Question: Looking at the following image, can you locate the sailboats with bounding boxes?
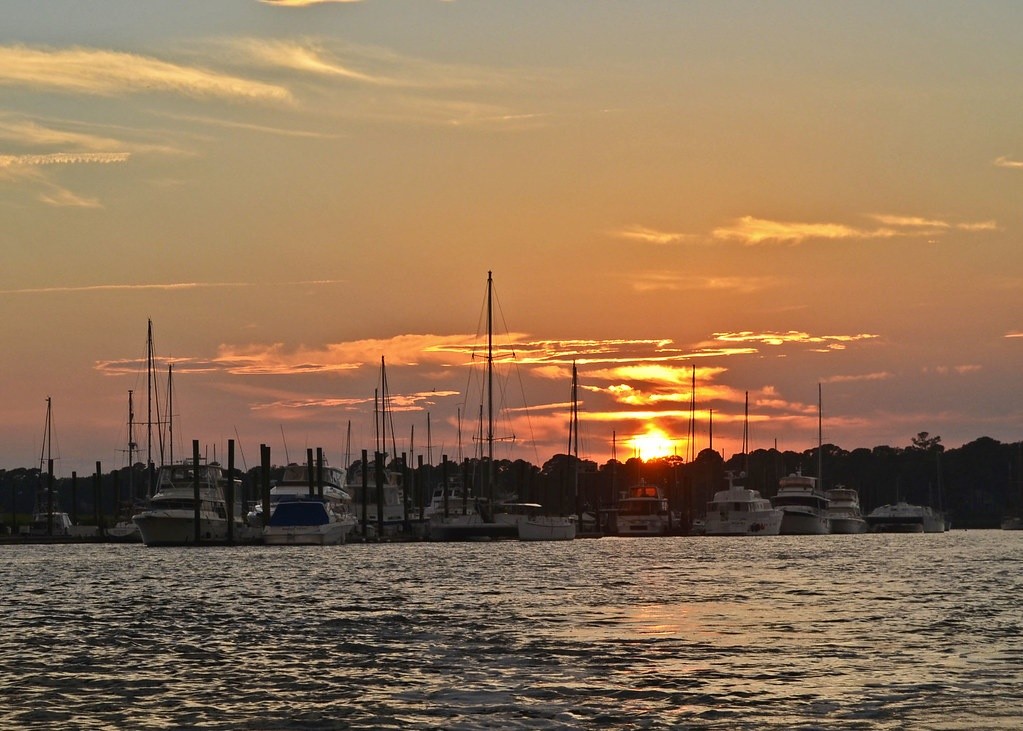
[104,270,950,548]
[19,397,99,536]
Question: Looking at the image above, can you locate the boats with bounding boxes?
[1001,516,1022,530]
